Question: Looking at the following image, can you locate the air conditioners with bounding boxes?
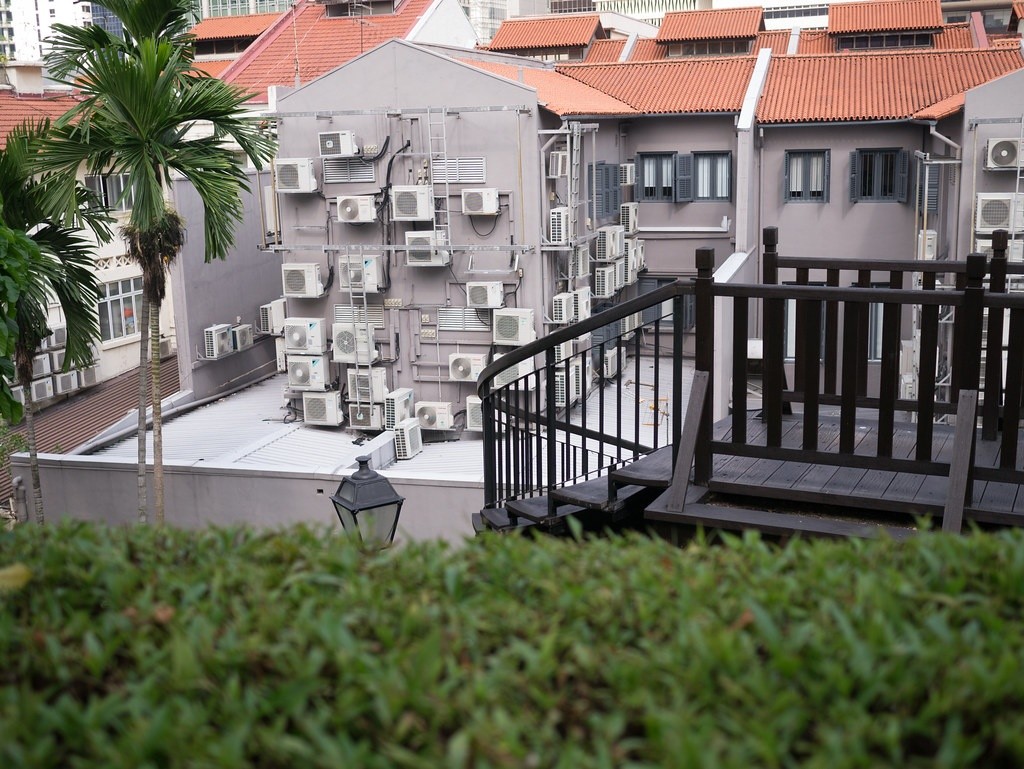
[899,191,1024,409]
[204,323,252,358]
[984,136,1023,168]
[4,323,103,406]
[159,337,172,360]
[336,195,378,223]
[258,301,384,431]
[390,184,435,221]
[264,158,317,237]
[461,187,501,215]
[404,230,450,266]
[385,281,535,459]
[281,262,324,300]
[339,254,385,293]
[317,131,357,158]
[544,150,647,409]
